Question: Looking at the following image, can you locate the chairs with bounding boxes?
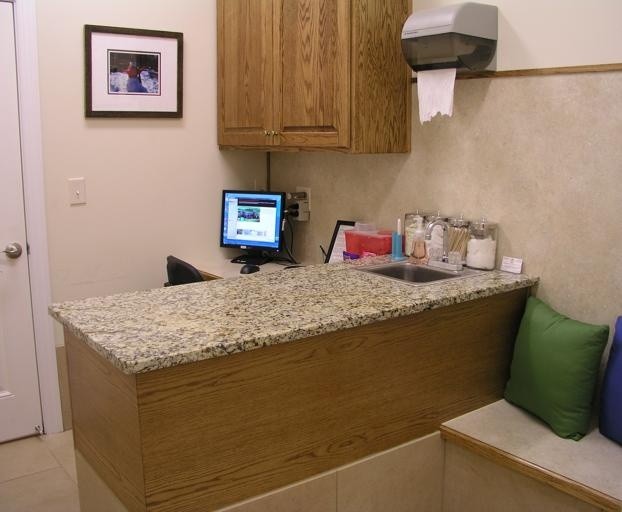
[166,256,204,283]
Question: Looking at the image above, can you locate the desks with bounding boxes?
[188,259,289,281]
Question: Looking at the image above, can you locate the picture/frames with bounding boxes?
[84,25,184,118]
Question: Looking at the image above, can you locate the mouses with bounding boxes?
[240,264,260,274]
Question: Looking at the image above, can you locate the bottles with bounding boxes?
[393,210,497,272]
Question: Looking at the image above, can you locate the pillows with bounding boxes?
[600,316,621,447]
[503,296,609,441]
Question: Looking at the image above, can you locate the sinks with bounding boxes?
[350,257,472,287]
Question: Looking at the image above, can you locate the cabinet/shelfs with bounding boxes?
[218,1,411,153]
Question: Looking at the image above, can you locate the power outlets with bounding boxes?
[294,189,311,225]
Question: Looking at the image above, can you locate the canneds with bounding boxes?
[405,210,497,271]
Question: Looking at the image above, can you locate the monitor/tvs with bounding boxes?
[220,190,286,265]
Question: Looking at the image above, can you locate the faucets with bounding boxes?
[424,217,464,272]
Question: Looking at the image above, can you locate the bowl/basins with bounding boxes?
[352,220,379,234]
